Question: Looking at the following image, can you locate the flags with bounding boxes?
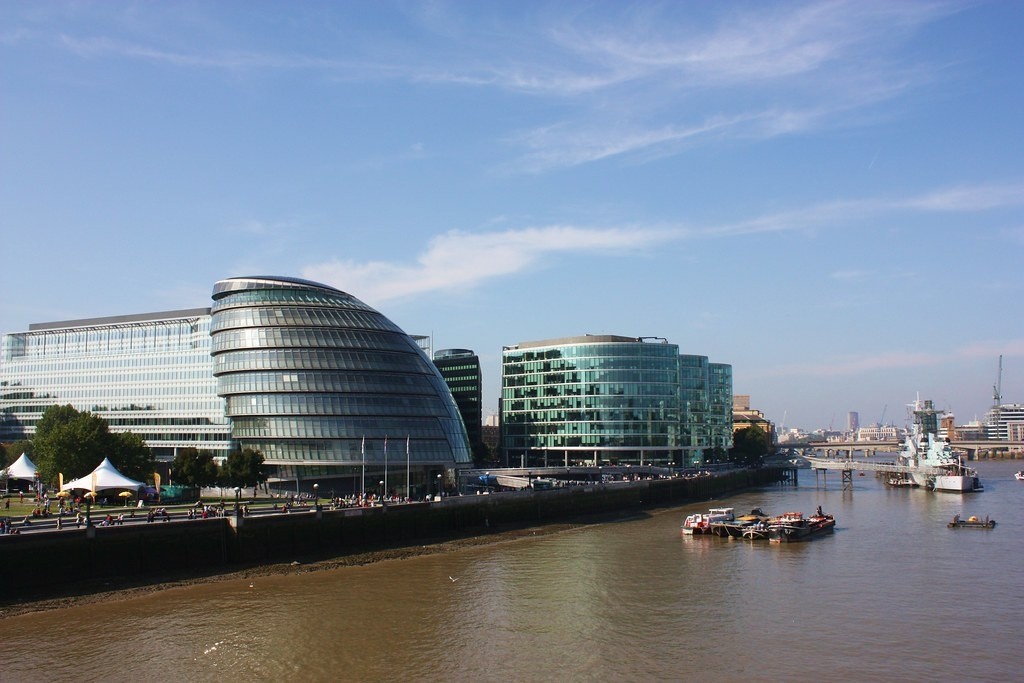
[406,436,410,454]
[361,437,365,454]
[383,437,387,454]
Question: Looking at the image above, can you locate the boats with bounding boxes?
[1014,470,1024,480]
[680,505,836,543]
[947,513,996,530]
[888,391,984,492]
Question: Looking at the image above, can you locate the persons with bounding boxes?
[0,486,530,535]
[539,461,711,487]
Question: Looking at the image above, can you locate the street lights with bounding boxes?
[528,470,532,488]
[599,466,602,482]
[668,462,675,475]
[437,473,442,495]
[313,484,319,510]
[234,487,240,512]
[627,465,631,479]
[648,463,652,476]
[486,472,489,491]
[86,493,91,528]
[379,481,385,504]
[694,455,763,471]
[567,468,570,484]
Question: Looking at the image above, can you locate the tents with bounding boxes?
[61,458,147,507]
[0,452,43,493]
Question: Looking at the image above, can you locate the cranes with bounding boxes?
[993,355,1002,405]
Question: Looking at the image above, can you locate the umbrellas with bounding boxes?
[84,492,98,505]
[56,492,70,507]
[141,487,167,506]
[118,491,133,507]
